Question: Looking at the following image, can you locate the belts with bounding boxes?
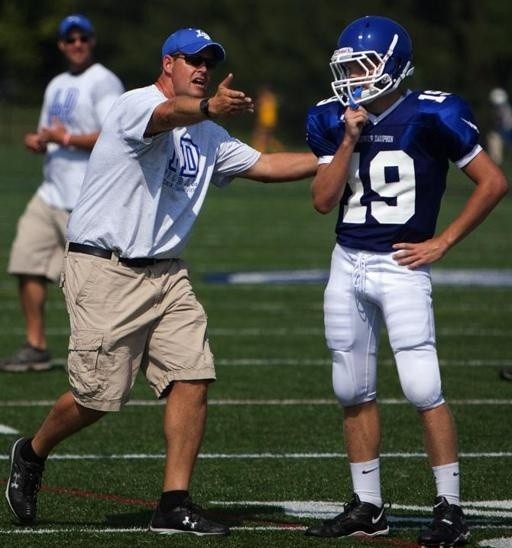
[68,241,166,268]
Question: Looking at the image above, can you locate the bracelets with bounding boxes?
[62,134,70,145]
[200,98,212,119]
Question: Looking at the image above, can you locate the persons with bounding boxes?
[303,15,509,546]
[4,25,316,535]
[487,87,511,169]
[0,15,128,373]
[251,87,284,156]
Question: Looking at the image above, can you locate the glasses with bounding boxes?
[176,54,216,69]
[65,37,89,43]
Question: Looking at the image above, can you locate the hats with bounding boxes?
[162,28,225,60]
[59,15,92,38]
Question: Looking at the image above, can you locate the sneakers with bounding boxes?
[6,435,45,521]
[305,493,392,540]
[418,497,471,548]
[1,342,52,372]
[150,496,231,537]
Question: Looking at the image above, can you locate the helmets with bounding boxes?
[329,16,416,108]
